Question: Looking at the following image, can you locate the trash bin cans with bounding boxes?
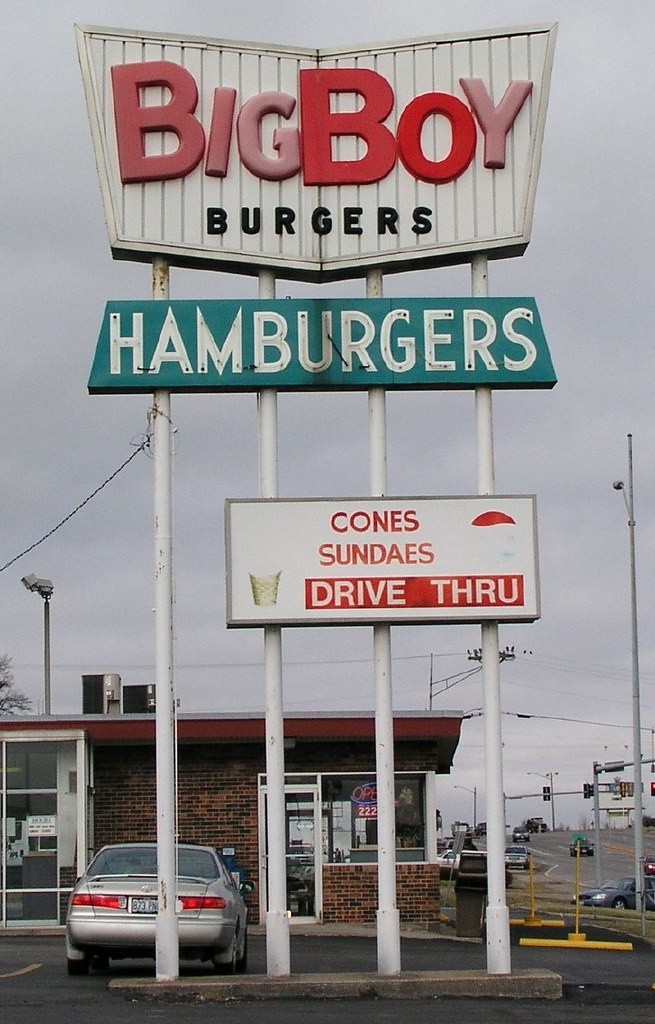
[452,856,486,937]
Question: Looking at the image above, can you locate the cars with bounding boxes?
[512,818,548,842]
[436,849,486,868]
[503,845,532,869]
[643,853,655,876]
[568,838,594,857]
[64,842,254,976]
[571,876,655,910]
[450,822,486,837]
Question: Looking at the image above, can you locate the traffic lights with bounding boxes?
[650,782,655,796]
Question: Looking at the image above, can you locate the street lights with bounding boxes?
[526,771,555,831]
[454,785,476,826]
[611,434,646,910]
